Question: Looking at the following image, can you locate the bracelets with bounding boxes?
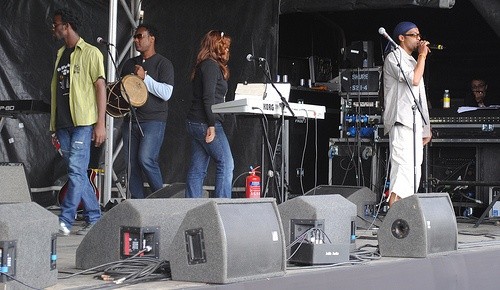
[418,53,426,59]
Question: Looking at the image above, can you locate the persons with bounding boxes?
[119,23,176,199]
[184,29,236,198]
[48,6,108,236]
[381,22,433,208]
[463,76,498,108]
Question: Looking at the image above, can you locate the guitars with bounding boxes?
[57,169,99,210]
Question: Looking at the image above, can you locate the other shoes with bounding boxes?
[76,223,95,235]
[59,222,72,236]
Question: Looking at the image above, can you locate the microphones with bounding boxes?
[426,44,447,50]
[246,53,265,62]
[378,26,399,49]
[96,36,110,45]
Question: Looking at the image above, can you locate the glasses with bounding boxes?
[133,33,151,38]
[402,32,422,38]
[52,22,66,28]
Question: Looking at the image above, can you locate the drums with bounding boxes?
[105,75,148,118]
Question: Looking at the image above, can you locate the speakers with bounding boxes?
[277,194,358,265]
[74,198,213,273]
[302,184,378,224]
[376,191,459,259]
[0,201,60,289]
[0,161,33,204]
[166,198,288,285]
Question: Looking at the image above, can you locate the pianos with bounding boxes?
[212,98,326,204]
[0,100,51,134]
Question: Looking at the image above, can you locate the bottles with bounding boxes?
[443,90,450,109]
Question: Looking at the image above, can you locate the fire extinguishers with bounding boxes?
[231,165,261,198]
